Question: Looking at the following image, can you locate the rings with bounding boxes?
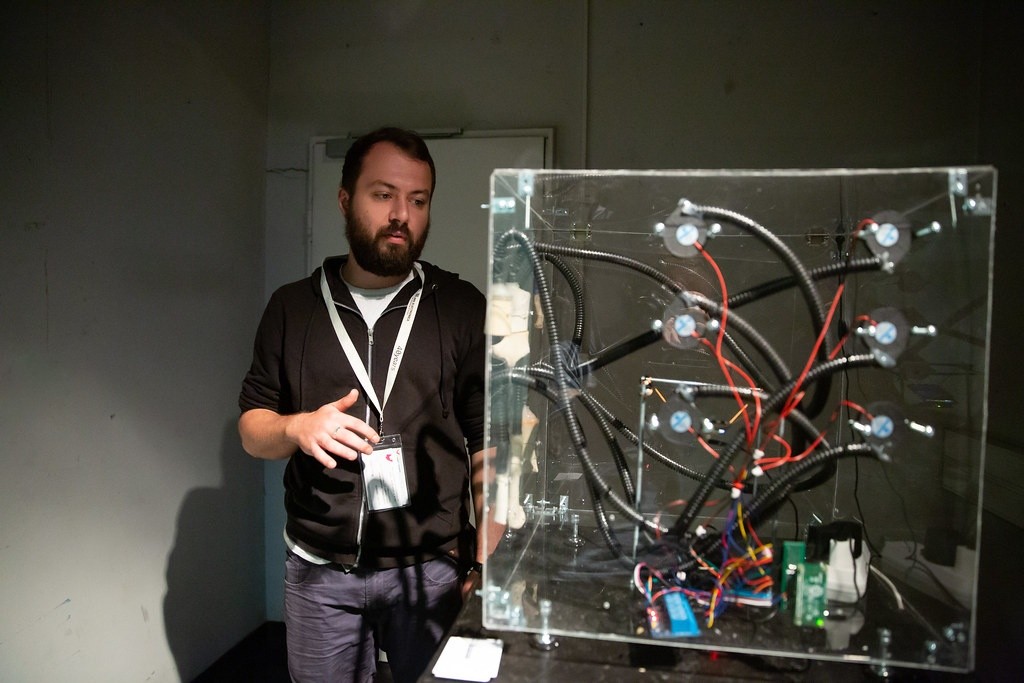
[334,426,340,439]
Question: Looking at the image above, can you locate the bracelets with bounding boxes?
[473,561,482,572]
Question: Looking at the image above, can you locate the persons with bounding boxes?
[238,128,507,683]
